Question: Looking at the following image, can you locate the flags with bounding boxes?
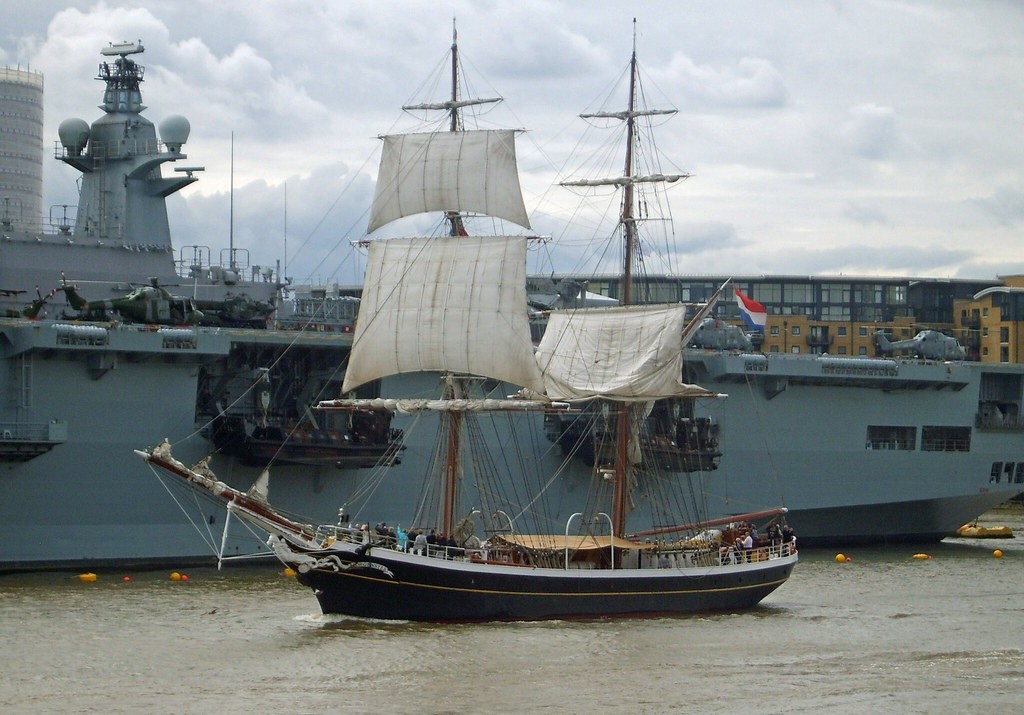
[734,285,767,335]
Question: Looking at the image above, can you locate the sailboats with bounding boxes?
[131,13,804,627]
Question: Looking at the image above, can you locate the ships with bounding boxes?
[0,19,1024,576]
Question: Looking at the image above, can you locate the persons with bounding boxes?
[720,520,797,566]
[353,521,466,559]
